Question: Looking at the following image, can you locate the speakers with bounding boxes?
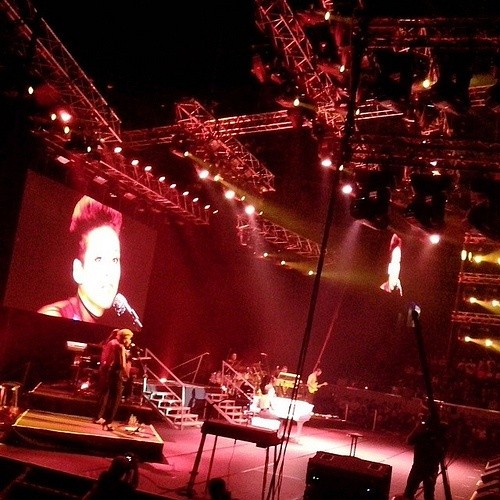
[305,451,392,500]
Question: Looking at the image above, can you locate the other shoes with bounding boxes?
[94,418,106,424]
[103,424,117,431]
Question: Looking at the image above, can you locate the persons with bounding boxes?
[94,330,133,431]
[38,195,120,325]
[402,411,448,500]
[224,352,321,406]
[379,234,408,299]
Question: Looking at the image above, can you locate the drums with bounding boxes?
[236,380,254,399]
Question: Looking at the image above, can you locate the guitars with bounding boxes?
[307,381,328,394]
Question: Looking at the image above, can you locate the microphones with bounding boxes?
[130,343,142,352]
[114,293,142,331]
[261,353,267,356]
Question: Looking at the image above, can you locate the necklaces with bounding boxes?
[77,291,103,322]
